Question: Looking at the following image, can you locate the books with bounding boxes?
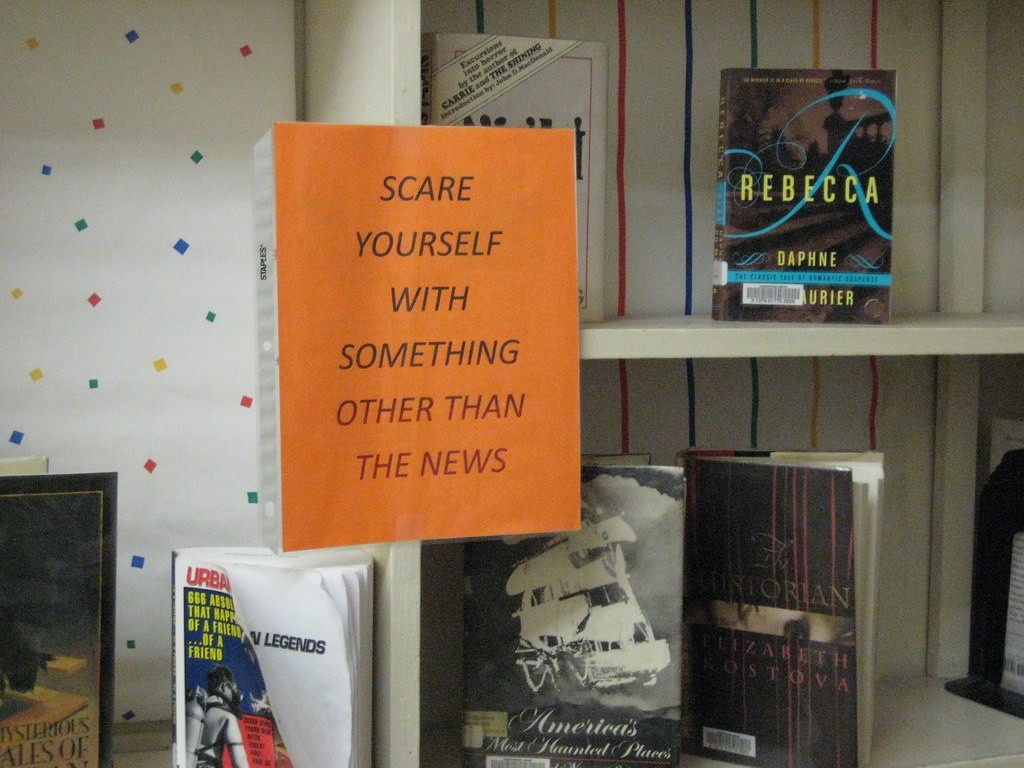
[458,453,685,768]
[420,31,604,321]
[711,69,897,325]
[172,547,374,768]
[675,450,885,768]
[0,457,118,768]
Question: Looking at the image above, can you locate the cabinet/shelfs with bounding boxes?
[296,1,1024,768]
